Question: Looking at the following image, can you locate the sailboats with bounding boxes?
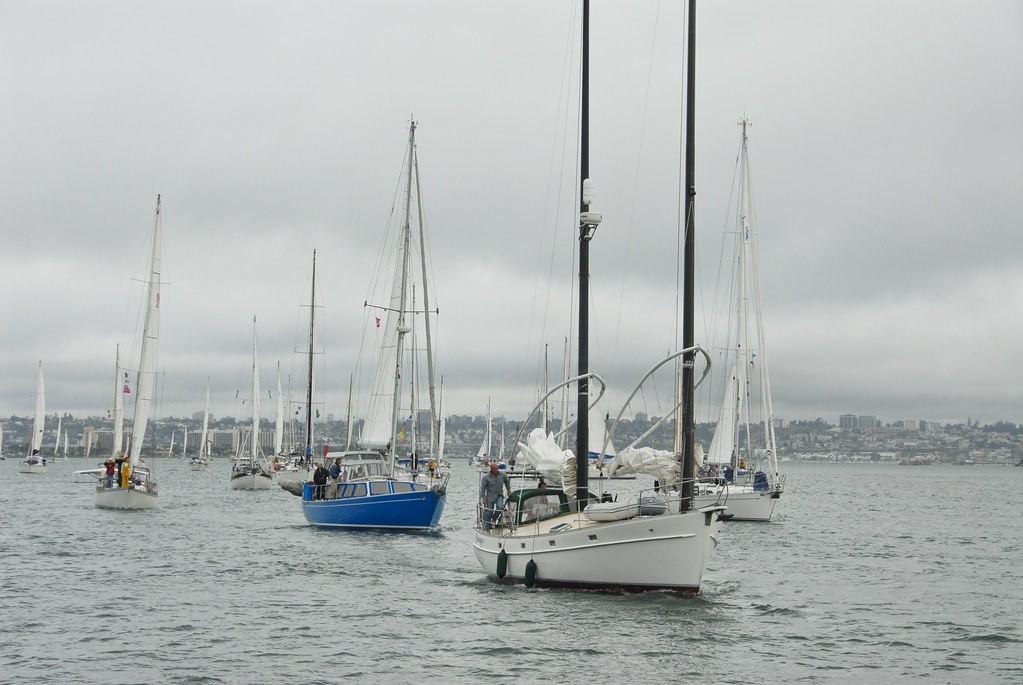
[301,112,455,533]
[43,416,94,464]
[95,195,167,511]
[19,359,47,474]
[229,314,299,492]
[474,0,734,596]
[655,113,785,522]
[271,246,335,499]
[177,428,188,462]
[468,395,512,469]
[190,376,213,472]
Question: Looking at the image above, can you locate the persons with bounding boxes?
[410,452,418,470]
[314,462,329,500]
[326,458,342,499]
[532,496,558,518]
[479,463,512,530]
[740,459,745,470]
[305,444,311,461]
[104,453,130,488]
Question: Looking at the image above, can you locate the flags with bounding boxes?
[316,410,320,418]
[376,317,380,328]
[124,385,130,393]
